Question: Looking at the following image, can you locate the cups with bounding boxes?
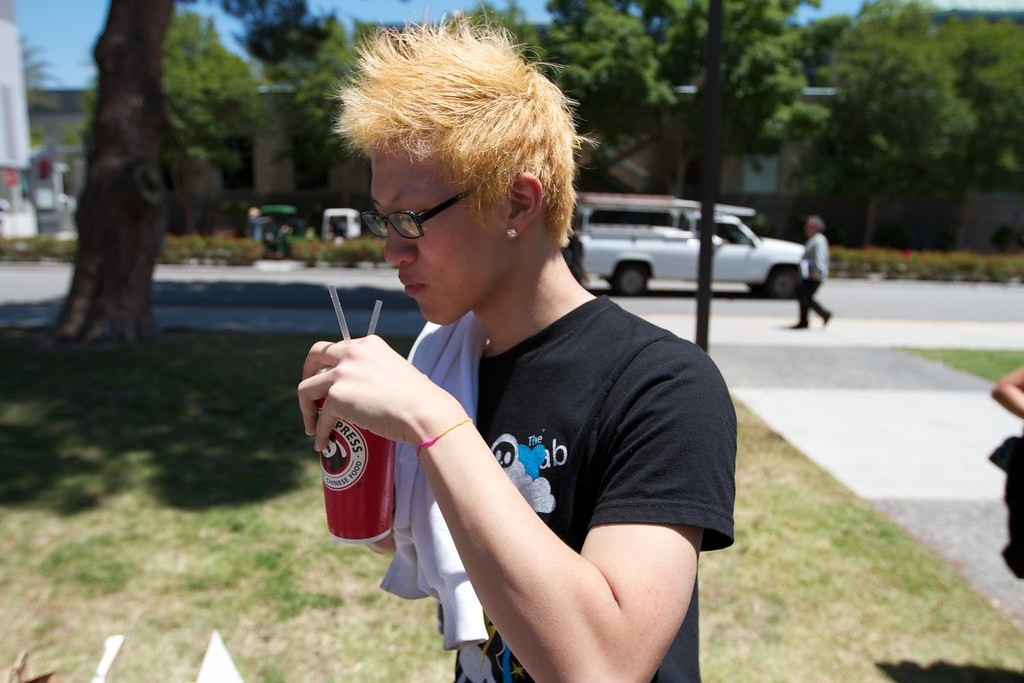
[313,367,396,543]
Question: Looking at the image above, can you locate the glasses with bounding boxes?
[361,188,480,240]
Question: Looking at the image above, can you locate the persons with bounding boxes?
[990,365,1024,418]
[792,215,833,329]
[296,9,739,683]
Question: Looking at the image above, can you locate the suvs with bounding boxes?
[566,193,811,297]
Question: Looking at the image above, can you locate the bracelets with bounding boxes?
[415,418,473,461]
